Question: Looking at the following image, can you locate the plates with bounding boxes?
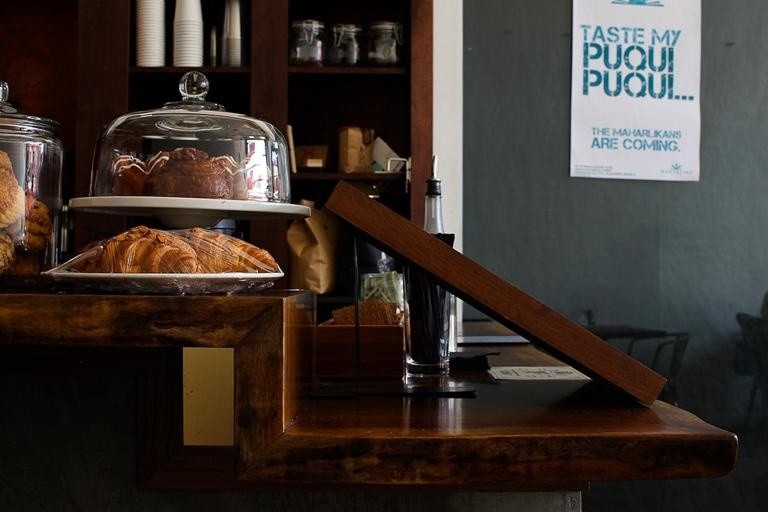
[41,266,289,296]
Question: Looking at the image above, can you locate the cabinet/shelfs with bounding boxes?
[75,1,432,304]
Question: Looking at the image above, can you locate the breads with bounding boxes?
[0,150,57,273]
[72,226,279,274]
[100,147,250,201]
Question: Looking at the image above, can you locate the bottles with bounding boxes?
[0,82,65,291]
[292,21,403,66]
[413,176,464,360]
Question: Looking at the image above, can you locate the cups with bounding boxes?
[135,1,243,69]
[401,263,452,378]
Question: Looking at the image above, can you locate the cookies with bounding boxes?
[333,301,401,325]
